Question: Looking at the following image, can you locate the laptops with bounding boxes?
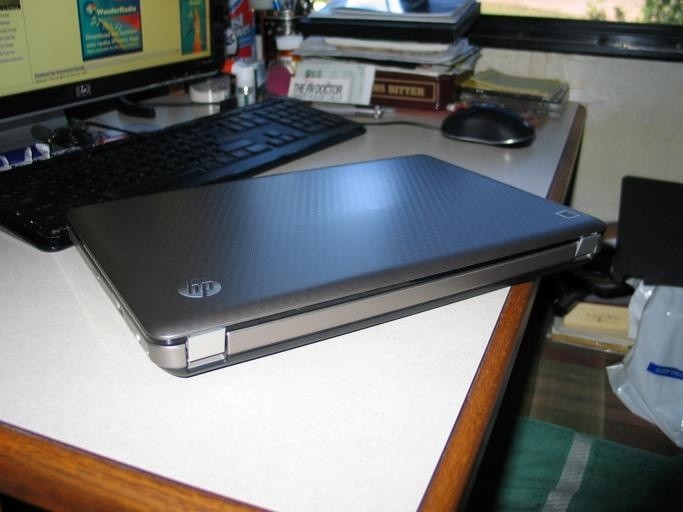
[64,152,607,379]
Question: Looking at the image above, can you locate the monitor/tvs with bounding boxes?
[0,0,227,149]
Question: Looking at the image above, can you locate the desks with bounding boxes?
[0,80,589,512]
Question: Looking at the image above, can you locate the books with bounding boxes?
[289,37,481,78]
[459,66,571,120]
[545,301,634,356]
[307,0,478,23]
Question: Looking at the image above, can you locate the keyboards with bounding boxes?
[0,95,367,253]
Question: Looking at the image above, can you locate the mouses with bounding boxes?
[439,105,537,150]
